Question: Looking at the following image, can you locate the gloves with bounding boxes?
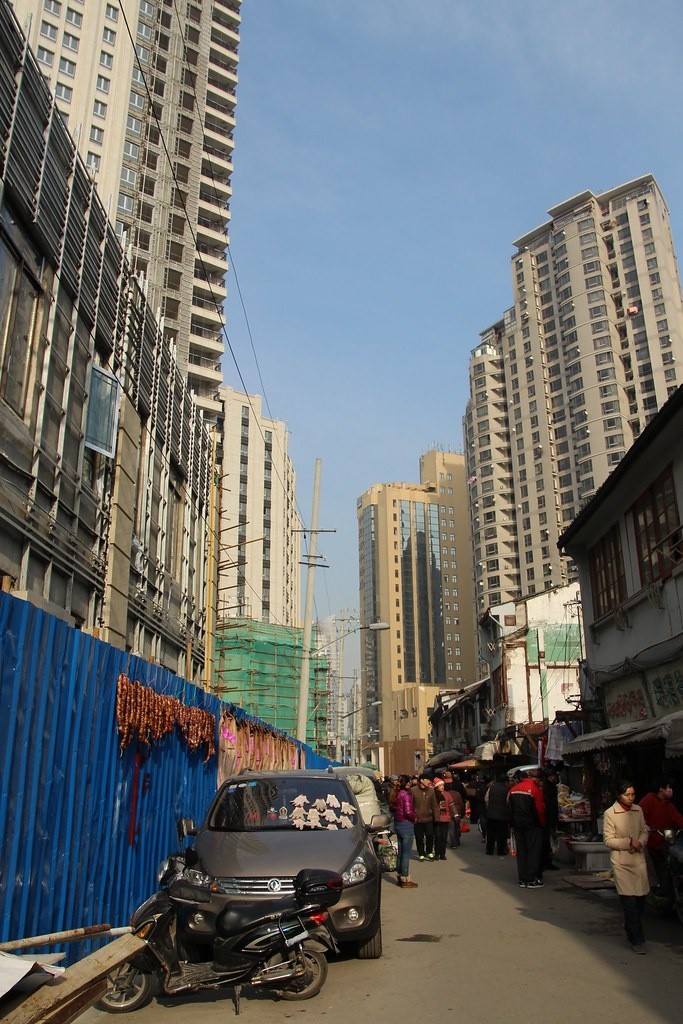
[455,816,460,824]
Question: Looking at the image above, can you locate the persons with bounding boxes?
[638,776,683,898]
[393,776,419,888]
[381,772,468,862]
[468,768,546,888]
[600,783,650,955]
[539,768,562,871]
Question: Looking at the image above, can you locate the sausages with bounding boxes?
[117,674,215,766]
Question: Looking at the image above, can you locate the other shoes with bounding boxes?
[435,855,439,860]
[527,879,544,888]
[631,944,647,953]
[427,853,433,858]
[440,856,446,860]
[419,856,425,859]
[481,837,486,843]
[518,881,527,887]
[546,864,559,870]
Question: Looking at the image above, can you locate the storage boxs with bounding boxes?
[569,840,611,871]
[598,819,603,834]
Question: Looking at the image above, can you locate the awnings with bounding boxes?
[561,709,683,768]
[447,757,495,769]
[474,740,511,761]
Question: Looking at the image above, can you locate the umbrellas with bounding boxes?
[424,748,464,769]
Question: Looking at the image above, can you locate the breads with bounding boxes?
[557,796,576,807]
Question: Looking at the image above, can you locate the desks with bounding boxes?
[560,817,593,835]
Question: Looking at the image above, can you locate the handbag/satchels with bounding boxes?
[550,836,560,857]
[459,818,471,833]
[507,830,518,857]
[465,782,477,797]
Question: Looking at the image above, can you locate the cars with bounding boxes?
[327,766,396,835]
[177,764,391,960]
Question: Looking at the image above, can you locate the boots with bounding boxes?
[397,875,400,886]
[400,875,418,888]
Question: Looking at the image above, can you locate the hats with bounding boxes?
[434,777,444,788]
[530,769,542,778]
[419,773,429,779]
[441,772,452,779]
[412,775,417,780]
[390,775,398,782]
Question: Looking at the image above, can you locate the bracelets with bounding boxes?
[630,838,633,846]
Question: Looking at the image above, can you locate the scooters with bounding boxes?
[95,846,346,1015]
[644,828,683,924]
